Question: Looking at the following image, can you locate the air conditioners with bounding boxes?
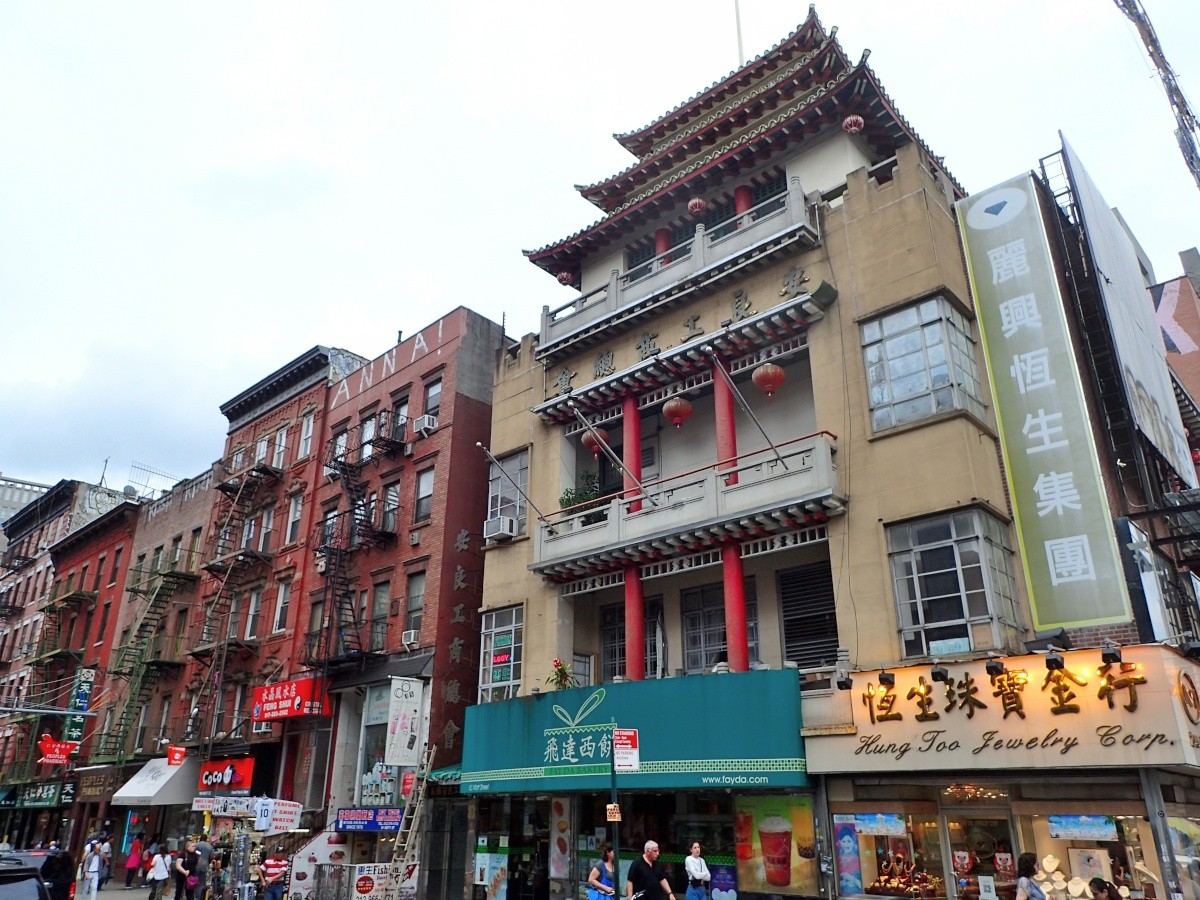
[414,414,436,433]
[484,516,518,540]
[253,721,272,733]
[402,630,419,645]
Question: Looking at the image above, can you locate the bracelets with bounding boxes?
[667,892,672,896]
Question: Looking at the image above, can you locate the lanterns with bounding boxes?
[842,112,864,135]
[687,197,706,217]
[662,397,692,432]
[751,364,785,400]
[582,428,607,460]
[557,272,573,285]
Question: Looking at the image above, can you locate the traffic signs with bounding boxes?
[613,729,640,771]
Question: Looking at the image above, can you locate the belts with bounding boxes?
[88,871,99,873]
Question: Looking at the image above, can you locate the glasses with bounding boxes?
[650,849,660,853]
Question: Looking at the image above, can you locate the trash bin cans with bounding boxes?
[239,884,255,900]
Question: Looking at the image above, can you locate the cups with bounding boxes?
[790,795,817,858]
[758,831,793,886]
[735,809,753,859]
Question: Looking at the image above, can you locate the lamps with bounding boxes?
[879,669,894,684]
[1045,649,1064,669]
[721,319,732,327]
[1102,643,1122,663]
[986,658,1003,675]
[837,674,852,690]
[650,348,661,356]
[931,663,948,682]
[1180,642,1200,656]
[564,387,573,394]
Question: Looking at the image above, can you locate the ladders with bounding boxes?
[380,742,439,900]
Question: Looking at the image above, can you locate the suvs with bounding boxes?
[0,849,76,900]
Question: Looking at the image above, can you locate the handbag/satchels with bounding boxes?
[146,867,156,883]
[142,849,149,861]
[186,876,200,890]
[137,865,144,877]
[690,879,702,887]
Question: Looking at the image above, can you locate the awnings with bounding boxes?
[111,756,201,806]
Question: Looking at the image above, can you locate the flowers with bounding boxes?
[545,658,580,691]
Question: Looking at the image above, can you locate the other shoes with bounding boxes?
[141,884,151,888]
[124,887,133,889]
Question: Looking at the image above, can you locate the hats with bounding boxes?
[276,846,285,852]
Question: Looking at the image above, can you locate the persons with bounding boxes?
[258,846,289,900]
[1087,878,1123,900]
[1015,852,1046,900]
[32,831,216,900]
[684,839,712,900]
[626,840,676,900]
[588,842,616,900]
[836,825,861,891]
